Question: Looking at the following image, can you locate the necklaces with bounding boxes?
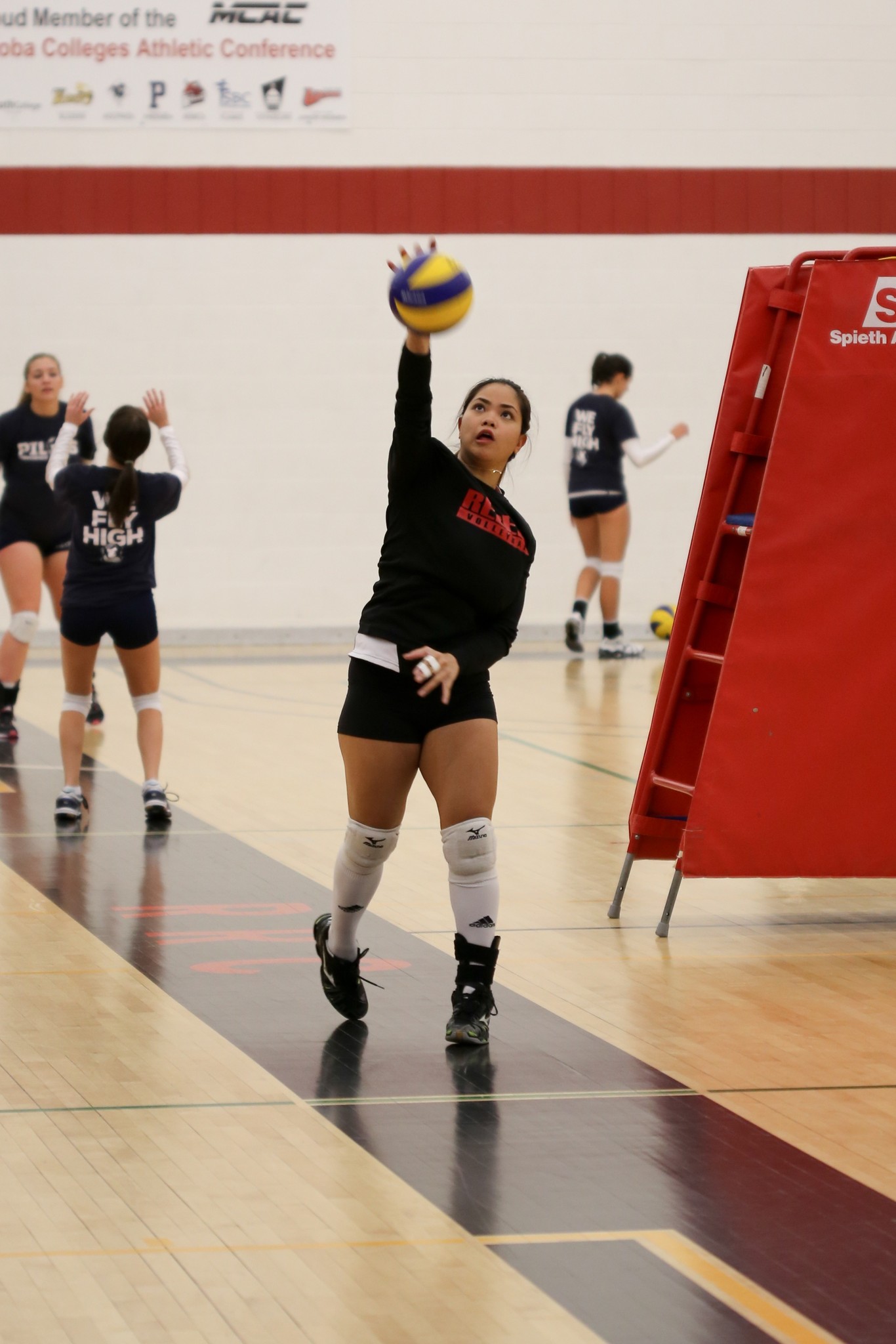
[468,465,503,475]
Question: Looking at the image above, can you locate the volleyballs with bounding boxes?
[650,604,677,640]
[389,250,473,334]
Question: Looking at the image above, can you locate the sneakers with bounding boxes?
[54,818,90,844]
[445,1042,499,1117]
[444,985,502,1045]
[564,609,586,654]
[313,913,385,1019]
[313,1019,369,1106]
[84,672,104,723]
[53,785,90,820]
[598,633,648,659]
[143,826,171,853]
[0,703,18,738]
[144,783,180,819]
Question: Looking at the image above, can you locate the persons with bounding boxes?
[46,388,187,821]
[564,352,689,660]
[313,237,538,1043]
[0,352,97,740]
